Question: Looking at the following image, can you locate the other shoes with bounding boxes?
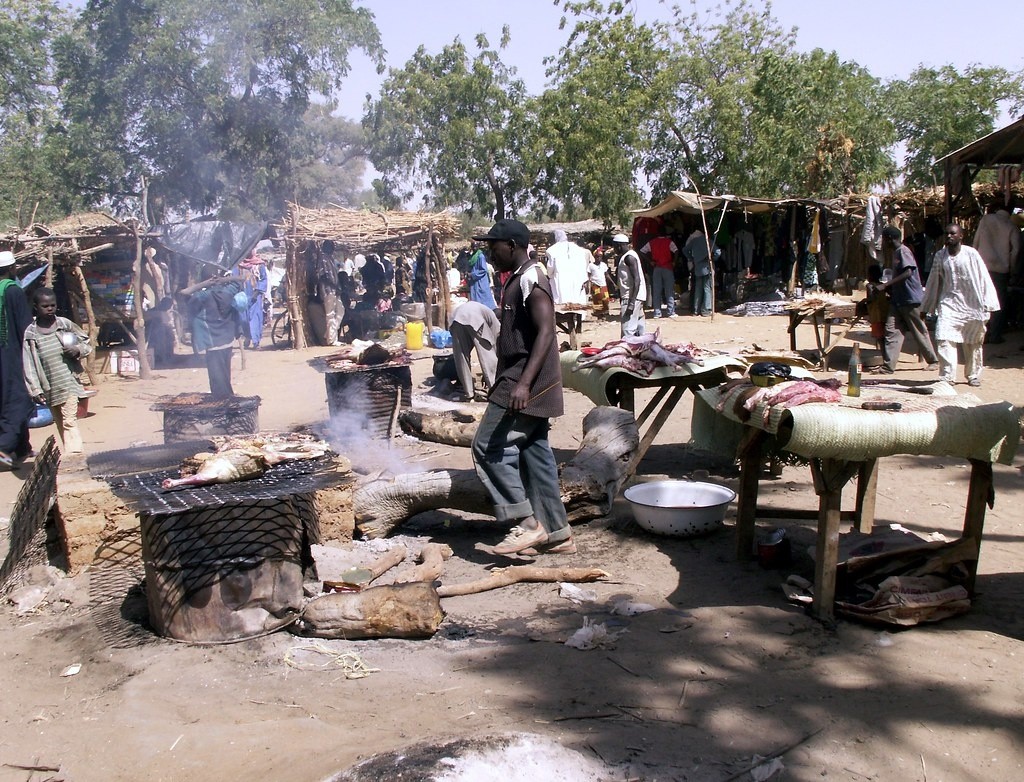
[668,314,678,317]
[702,310,713,317]
[925,363,938,371]
[654,313,662,319]
[871,366,893,375]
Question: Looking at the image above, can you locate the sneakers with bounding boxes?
[516,535,577,555]
[491,520,549,555]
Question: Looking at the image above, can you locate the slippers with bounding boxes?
[0,456,21,469]
[18,450,38,461]
[948,380,956,386]
[968,378,980,387]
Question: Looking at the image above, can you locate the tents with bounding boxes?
[629,191,866,322]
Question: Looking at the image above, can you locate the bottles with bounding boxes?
[766,527,785,543]
[847,343,863,397]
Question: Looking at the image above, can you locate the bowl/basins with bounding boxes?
[623,479,736,535]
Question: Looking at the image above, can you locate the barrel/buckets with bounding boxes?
[405,321,425,350]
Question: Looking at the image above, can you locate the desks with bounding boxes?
[568,339,801,493]
[717,391,1014,628]
[782,298,922,372]
[556,304,595,349]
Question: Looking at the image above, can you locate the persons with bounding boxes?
[449,300,502,399]
[0,251,34,469]
[22,287,92,452]
[231,247,268,350]
[144,296,176,356]
[863,265,890,353]
[613,232,646,339]
[639,227,679,319]
[869,227,939,375]
[920,223,1001,386]
[527,229,619,334]
[973,204,1021,345]
[682,229,721,317]
[472,219,577,555]
[180,265,246,394]
[316,239,498,346]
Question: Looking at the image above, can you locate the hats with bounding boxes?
[471,219,530,248]
[0,251,15,267]
[613,234,629,242]
[593,249,602,256]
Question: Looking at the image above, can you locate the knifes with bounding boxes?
[822,402,902,409]
[861,385,933,394]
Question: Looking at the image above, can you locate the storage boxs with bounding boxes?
[111,345,154,373]
[77,271,134,313]
